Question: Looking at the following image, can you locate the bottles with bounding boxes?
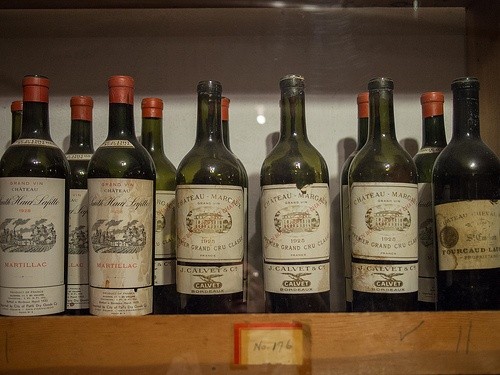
[0,72,500,317]
